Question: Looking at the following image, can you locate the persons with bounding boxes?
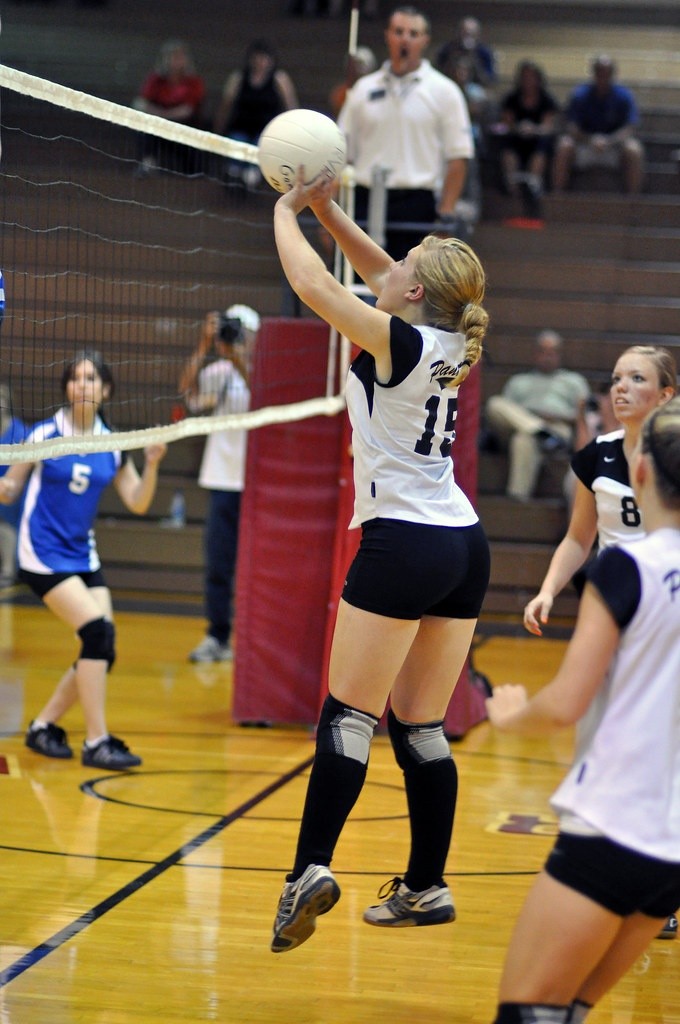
[270,164,491,954]
[132,16,645,240]
[318,6,475,308]
[484,324,680,1024]
[0,237,346,771]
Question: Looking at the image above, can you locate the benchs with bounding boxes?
[0,79,680,619]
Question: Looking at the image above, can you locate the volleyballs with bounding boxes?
[259,107,349,194]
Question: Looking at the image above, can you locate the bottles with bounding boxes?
[170,487,186,529]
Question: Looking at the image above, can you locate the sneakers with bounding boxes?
[364,877,455,927]
[80,733,142,768]
[657,914,677,938]
[25,720,73,758]
[270,864,340,953]
[190,636,233,663]
